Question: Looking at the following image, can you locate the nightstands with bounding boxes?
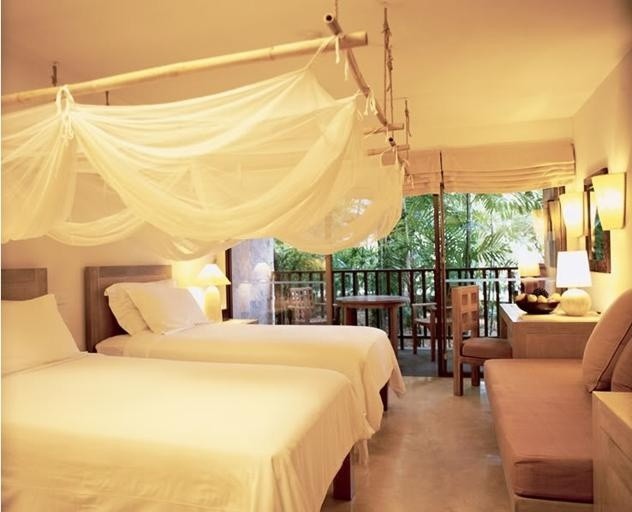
[222,316,260,325]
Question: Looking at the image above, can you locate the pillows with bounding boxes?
[581,284,631,391]
[4,293,81,378]
[610,336,631,390]
[103,280,180,336]
[122,280,209,334]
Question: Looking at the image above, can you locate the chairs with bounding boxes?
[451,285,511,396]
[287,287,341,324]
[410,283,460,360]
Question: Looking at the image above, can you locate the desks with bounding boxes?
[334,295,412,362]
[499,303,604,359]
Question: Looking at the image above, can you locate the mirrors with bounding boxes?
[583,168,610,273]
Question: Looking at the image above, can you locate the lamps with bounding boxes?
[589,172,625,230]
[557,192,583,238]
[518,251,545,294]
[554,250,593,316]
[530,207,551,245]
[176,264,233,324]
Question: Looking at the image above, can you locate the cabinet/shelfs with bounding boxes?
[591,389,631,511]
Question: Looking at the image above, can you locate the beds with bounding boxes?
[2,266,375,510]
[84,263,407,465]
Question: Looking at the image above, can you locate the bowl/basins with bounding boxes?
[514,301,560,315]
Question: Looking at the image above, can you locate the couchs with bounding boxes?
[482,286,632,511]
